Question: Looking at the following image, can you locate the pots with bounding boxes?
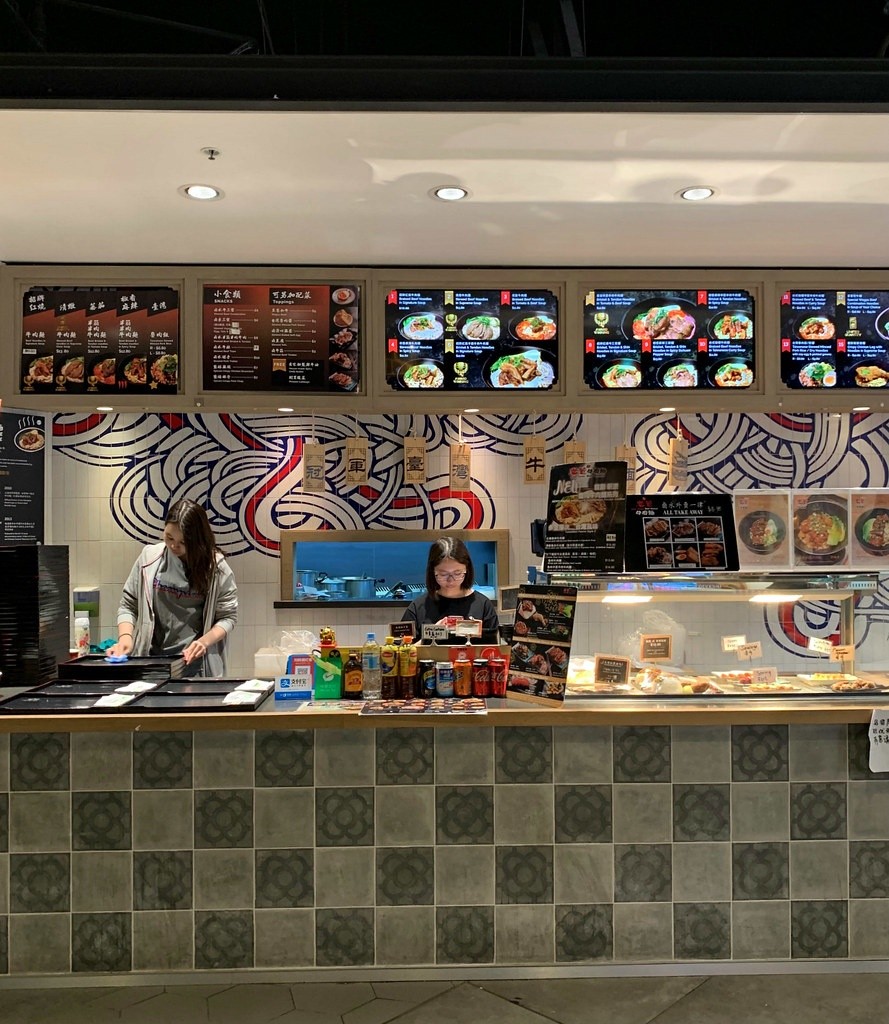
[297,570,385,600]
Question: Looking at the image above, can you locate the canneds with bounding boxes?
[416,658,508,698]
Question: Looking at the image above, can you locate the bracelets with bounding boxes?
[118,633,133,641]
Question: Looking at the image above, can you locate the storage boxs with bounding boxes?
[252,647,289,678]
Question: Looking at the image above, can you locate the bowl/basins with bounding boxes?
[595,358,641,388]
[874,307,889,341]
[794,500,848,557]
[854,507,889,556]
[792,310,836,341]
[846,358,889,388]
[508,310,557,341]
[707,309,754,339]
[707,356,754,387]
[481,345,558,388]
[739,510,786,556]
[23,352,179,390]
[798,360,836,387]
[621,296,697,340]
[656,357,698,387]
[398,311,445,341]
[455,310,502,341]
[396,358,444,389]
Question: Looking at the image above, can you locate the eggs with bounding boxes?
[822,372,837,387]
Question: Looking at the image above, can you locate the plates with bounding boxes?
[329,372,357,391]
[797,673,859,686]
[14,427,46,453]
[330,328,357,350]
[332,288,355,305]
[334,314,353,327]
[329,352,357,369]
[511,642,534,662]
[545,646,568,670]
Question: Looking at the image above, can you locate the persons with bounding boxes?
[401,537,499,645]
[106,499,240,678]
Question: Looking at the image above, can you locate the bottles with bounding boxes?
[361,632,382,700]
[342,654,364,700]
[398,636,418,699]
[380,636,399,700]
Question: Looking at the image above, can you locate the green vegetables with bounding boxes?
[156,354,178,374]
[802,317,830,327]
[763,518,778,547]
[827,516,846,546]
[806,361,835,378]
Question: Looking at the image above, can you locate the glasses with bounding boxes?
[434,569,467,581]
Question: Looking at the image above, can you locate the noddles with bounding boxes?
[602,304,754,387]
[403,313,557,389]
